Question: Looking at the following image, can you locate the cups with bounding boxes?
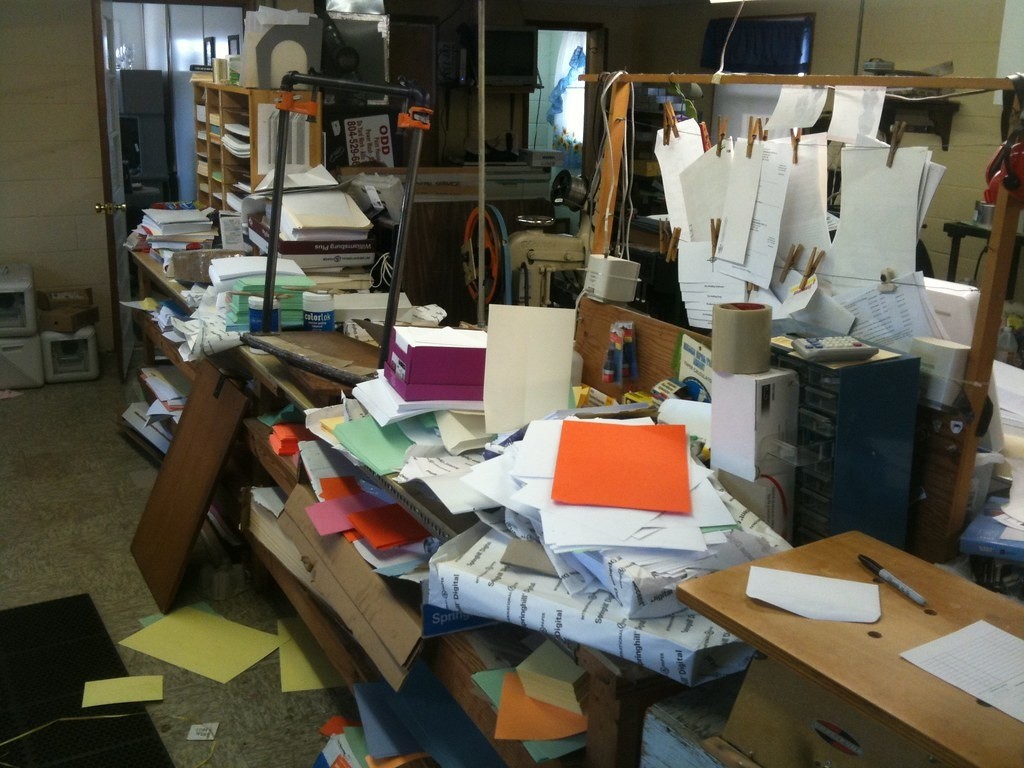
[212,58,227,83]
[711,302,771,375]
[228,55,241,85]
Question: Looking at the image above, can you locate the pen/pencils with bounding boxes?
[856,553,928,606]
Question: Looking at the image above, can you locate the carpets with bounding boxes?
[0,593,177,768]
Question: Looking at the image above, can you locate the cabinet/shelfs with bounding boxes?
[127,245,683,768]
[631,294,921,547]
[194,80,322,210]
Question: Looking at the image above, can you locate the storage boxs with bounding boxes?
[383,324,488,401]
[36,286,100,332]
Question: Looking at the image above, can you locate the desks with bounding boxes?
[675,529,1024,768]
[944,219,1024,300]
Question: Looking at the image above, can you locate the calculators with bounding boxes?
[788,335,880,363]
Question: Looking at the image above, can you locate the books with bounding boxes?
[516,639,590,718]
[122,100,373,429]
